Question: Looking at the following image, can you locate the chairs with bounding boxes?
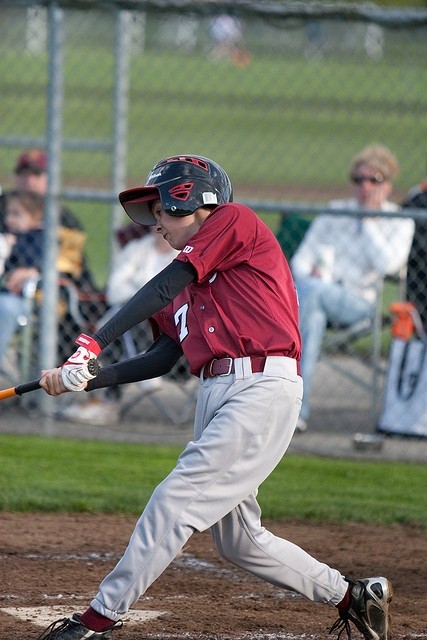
[273,212,388,413]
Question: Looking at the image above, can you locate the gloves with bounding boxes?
[61,333,102,392]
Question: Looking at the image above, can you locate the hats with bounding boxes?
[352,146,396,178]
[15,153,47,175]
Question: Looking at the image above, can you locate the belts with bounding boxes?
[203,357,302,380]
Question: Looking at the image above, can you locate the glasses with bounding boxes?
[351,176,384,185]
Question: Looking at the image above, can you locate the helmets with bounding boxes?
[119,155,232,226]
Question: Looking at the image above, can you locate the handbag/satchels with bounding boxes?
[378,340,426,436]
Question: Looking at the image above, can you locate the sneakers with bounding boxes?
[329,574,393,640]
[37,613,123,640]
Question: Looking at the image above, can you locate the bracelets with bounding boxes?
[75,333,101,356]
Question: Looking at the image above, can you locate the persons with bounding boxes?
[34,154,394,640]
[0,147,124,429]
[290,141,417,432]
[105,218,201,425]
[0,188,96,335]
